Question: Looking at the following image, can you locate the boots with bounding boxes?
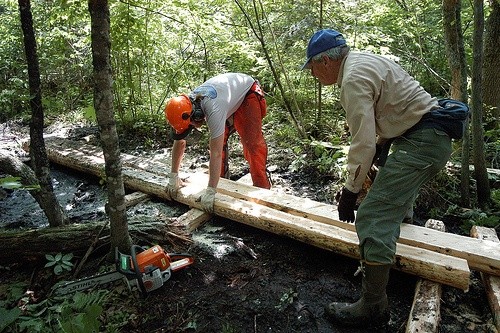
[326,260,391,325]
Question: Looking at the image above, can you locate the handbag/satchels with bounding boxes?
[432,98,469,140]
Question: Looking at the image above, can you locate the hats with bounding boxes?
[301,29,346,71]
[165,93,196,140]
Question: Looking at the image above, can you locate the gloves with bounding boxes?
[370,143,383,166]
[164,173,187,199]
[335,186,359,223]
[195,187,217,214]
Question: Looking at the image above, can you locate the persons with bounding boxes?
[301,28,452,323]
[165,72,272,214]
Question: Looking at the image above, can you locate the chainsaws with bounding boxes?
[53,244,193,297]
